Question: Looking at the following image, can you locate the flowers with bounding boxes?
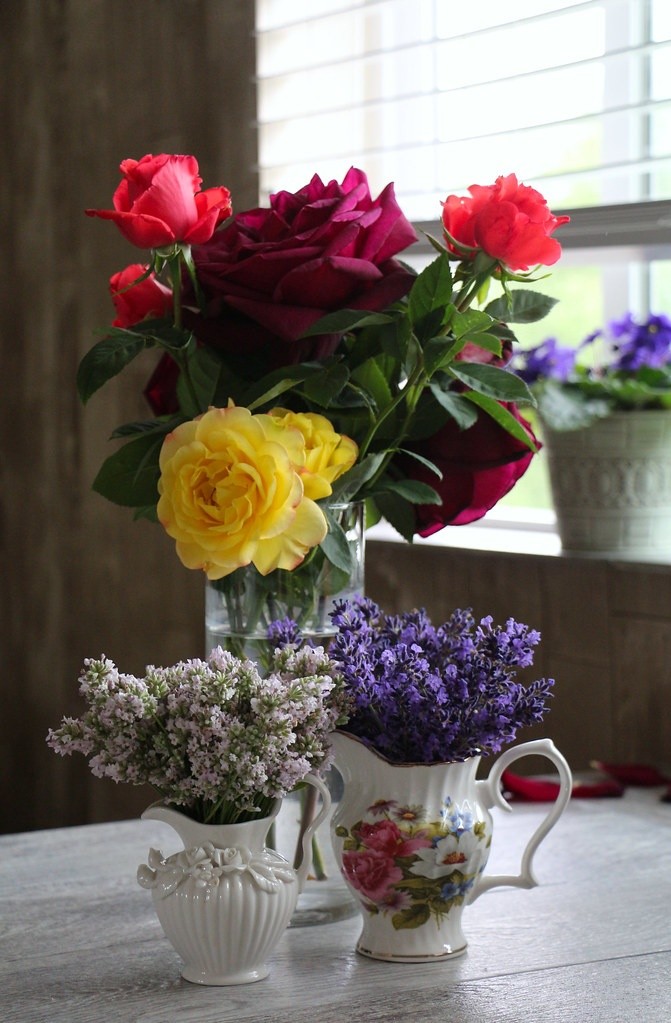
[507,313,671,430]
[77,155,568,875]
[265,596,554,766]
[47,643,350,823]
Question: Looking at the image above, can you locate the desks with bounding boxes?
[0,769,670,1023]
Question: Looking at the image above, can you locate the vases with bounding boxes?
[136,775,330,988]
[204,500,372,928]
[317,730,572,963]
[546,410,671,552]
